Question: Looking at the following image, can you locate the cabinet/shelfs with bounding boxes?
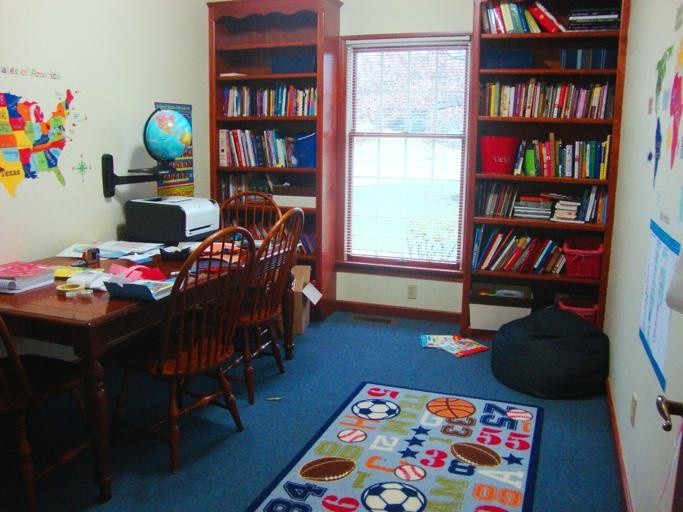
[458,1,632,341]
[206,0,342,322]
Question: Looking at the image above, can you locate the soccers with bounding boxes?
[353,398,400,420]
[361,482,427,512]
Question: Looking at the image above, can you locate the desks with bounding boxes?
[0,240,305,502]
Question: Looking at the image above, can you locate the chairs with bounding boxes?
[114,225,260,475]
[227,206,306,405]
[219,192,283,245]
[2,318,115,510]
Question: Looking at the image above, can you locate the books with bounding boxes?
[514,132,613,179]
[421,334,462,349]
[472,281,532,299]
[0,219,316,303]
[218,175,316,208]
[222,82,317,117]
[481,4,621,34]
[479,77,614,120]
[217,127,315,169]
[472,181,609,275]
[441,337,490,357]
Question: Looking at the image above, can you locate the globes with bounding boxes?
[143,108,192,175]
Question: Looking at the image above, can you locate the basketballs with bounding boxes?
[429,398,475,420]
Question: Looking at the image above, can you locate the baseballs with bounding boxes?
[507,409,533,422]
[395,464,426,481]
[338,428,368,443]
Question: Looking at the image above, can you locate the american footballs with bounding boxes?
[452,442,500,466]
[298,457,355,481]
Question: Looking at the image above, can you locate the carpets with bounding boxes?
[244,381,544,511]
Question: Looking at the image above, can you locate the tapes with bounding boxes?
[65,289,94,298]
[55,283,85,297]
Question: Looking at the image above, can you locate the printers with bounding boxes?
[125,196,220,243]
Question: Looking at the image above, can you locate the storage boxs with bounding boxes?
[288,265,322,335]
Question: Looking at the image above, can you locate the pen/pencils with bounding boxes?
[170,269,205,276]
